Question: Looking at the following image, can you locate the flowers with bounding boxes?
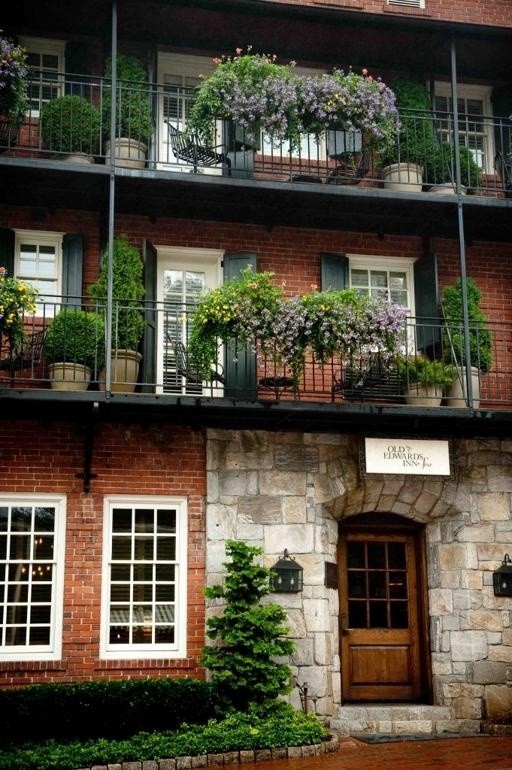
[186,46,403,154]
[1,30,32,125]
[0,266,42,345]
[178,264,408,380]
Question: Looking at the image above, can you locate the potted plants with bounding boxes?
[393,276,494,409]
[381,60,482,195]
[47,231,148,393]
[38,41,156,169]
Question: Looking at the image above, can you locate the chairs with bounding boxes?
[326,145,375,184]
[0,328,48,388]
[164,119,231,178]
[174,339,366,403]
